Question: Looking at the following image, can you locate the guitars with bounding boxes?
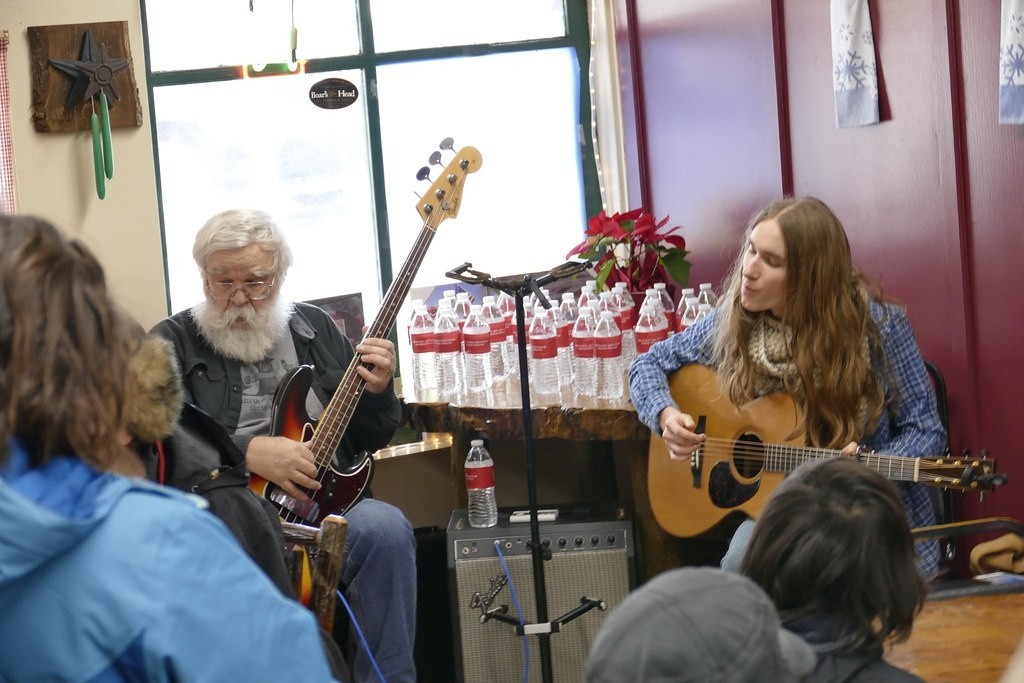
[243,137,483,609]
[645,362,1011,536]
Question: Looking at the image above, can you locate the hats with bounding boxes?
[582,564,818,683]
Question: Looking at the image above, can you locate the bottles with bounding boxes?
[465,439,498,528]
[405,277,720,408]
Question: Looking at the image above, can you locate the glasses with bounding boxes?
[205,267,277,300]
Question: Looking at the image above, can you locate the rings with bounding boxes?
[377,366,395,376]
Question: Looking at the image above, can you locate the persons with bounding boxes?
[627,195,946,598]
[581,567,821,683]
[1,211,340,683]
[741,456,930,683]
[137,207,419,683]
